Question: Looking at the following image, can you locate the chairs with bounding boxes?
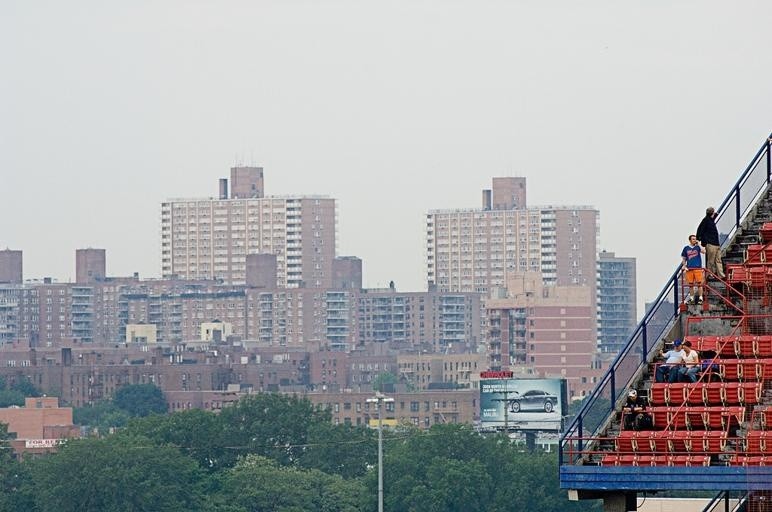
[600,222,771,466]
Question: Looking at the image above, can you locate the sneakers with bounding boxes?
[685,298,703,305]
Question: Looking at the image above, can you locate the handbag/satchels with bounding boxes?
[702,359,719,372]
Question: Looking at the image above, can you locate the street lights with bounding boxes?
[365,389,395,512]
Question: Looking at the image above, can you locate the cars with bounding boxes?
[508,389,558,413]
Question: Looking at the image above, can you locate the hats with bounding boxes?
[628,389,638,397]
[706,207,715,214]
[672,340,682,347]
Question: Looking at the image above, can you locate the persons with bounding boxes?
[656,340,685,384]
[678,341,701,383]
[622,389,648,430]
[695,205,727,280]
[680,234,707,305]
